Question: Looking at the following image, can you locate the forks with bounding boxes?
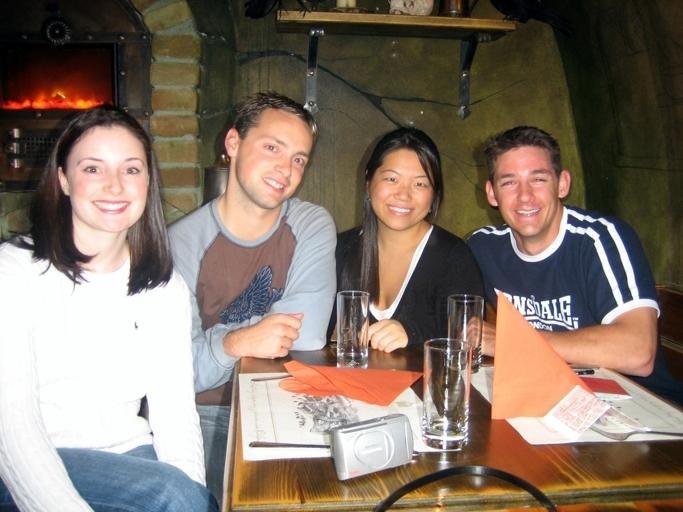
[589,425,683,441]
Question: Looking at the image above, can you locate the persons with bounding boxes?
[318,124,483,359]
[0,104,219,512]
[137,89,338,505]
[447,125,683,413]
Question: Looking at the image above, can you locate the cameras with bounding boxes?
[330,413,414,481]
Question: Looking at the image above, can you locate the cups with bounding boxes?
[336,290,369,370]
[447,294,484,374]
[423,338,472,451]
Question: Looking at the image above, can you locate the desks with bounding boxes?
[217,326,683,512]
